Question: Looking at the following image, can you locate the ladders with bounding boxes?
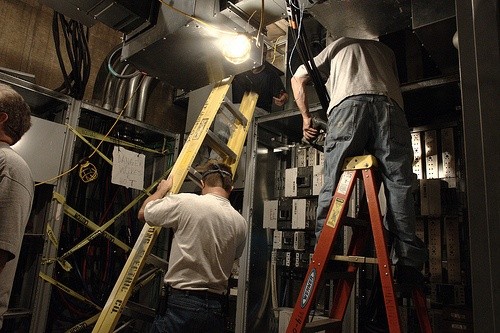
[285,154,432,333]
[91,75,260,333]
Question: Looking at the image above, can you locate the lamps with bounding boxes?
[221,32,252,66]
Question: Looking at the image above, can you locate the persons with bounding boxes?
[137,160,249,333]
[291,36,432,291]
[0,81,36,332]
[231,41,289,146]
